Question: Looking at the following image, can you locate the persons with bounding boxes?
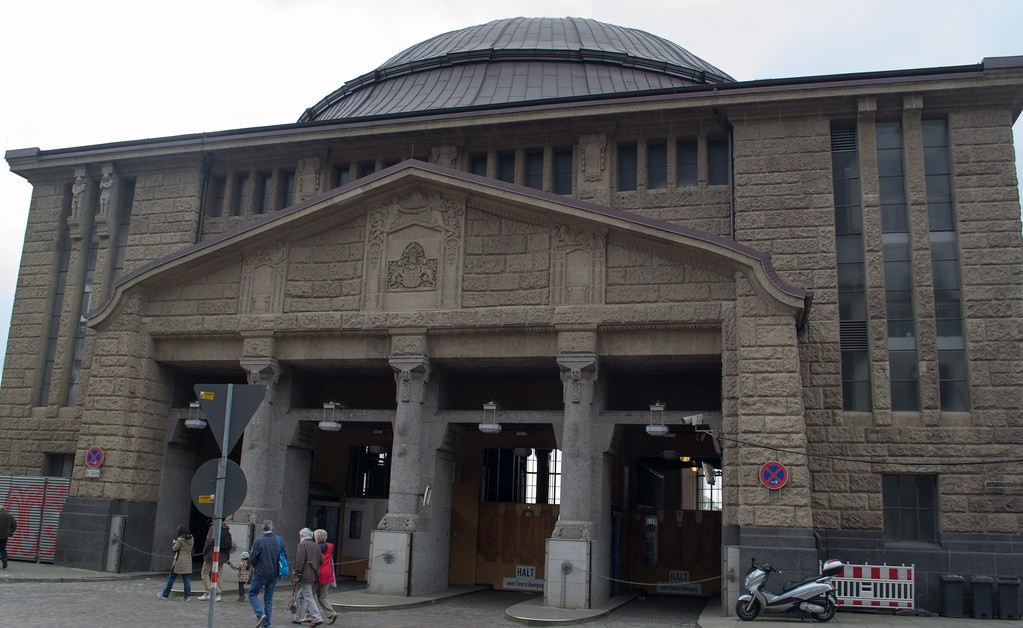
[223,550,253,604]
[291,527,325,627]
[197,510,232,602]
[69,175,88,220]
[245,519,287,627]
[1,505,18,567]
[156,523,196,601]
[300,528,337,627]
[99,173,114,217]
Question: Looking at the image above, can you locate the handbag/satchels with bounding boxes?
[275,535,291,576]
[330,557,338,588]
[288,599,298,615]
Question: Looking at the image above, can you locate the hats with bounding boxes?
[240,552,249,560]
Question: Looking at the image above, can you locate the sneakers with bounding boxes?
[253,614,271,628]
[196,593,221,602]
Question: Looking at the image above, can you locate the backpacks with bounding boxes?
[219,524,232,550]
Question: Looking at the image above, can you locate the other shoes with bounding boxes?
[310,620,323,628]
[236,596,246,602]
[156,592,168,601]
[291,620,301,625]
[326,614,337,625]
[181,596,192,602]
[300,616,313,622]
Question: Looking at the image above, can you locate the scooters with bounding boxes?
[732,555,843,623]
